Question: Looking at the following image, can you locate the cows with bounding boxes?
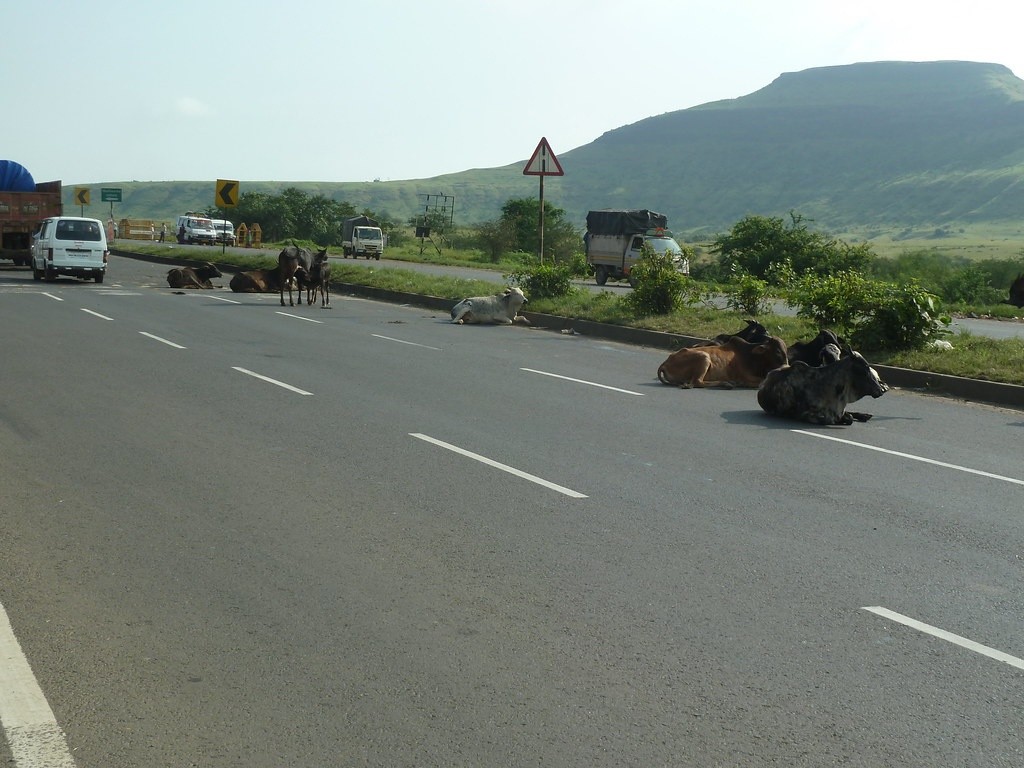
[655,319,889,425]
[165,238,332,308]
[452,286,532,326]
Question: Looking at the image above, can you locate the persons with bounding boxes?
[151,224,155,241]
[155,222,167,243]
[114,222,119,240]
[180,223,186,244]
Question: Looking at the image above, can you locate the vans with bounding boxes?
[31,217,109,284]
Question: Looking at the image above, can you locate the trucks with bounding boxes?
[175,213,216,245]
[584,208,689,288]
[210,219,235,246]
[0,180,63,266]
[340,216,384,260]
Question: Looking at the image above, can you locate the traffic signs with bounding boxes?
[101,187,122,202]
